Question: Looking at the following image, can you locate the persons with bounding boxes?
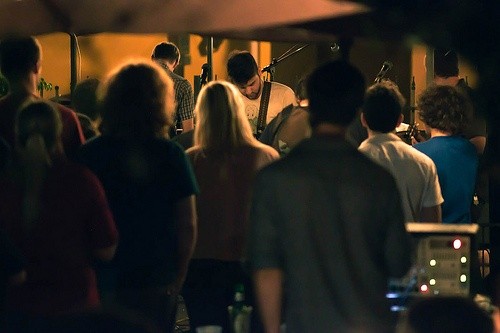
[77,60,200,333]
[151,42,195,137]
[357,81,445,223]
[426,40,489,153]
[226,49,297,138]
[411,84,492,292]
[0,101,118,333]
[246,59,409,333]
[259,73,312,155]
[0,35,85,155]
[68,78,104,140]
[179,80,282,333]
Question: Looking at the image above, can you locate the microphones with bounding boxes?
[373,60,394,83]
[328,41,340,52]
